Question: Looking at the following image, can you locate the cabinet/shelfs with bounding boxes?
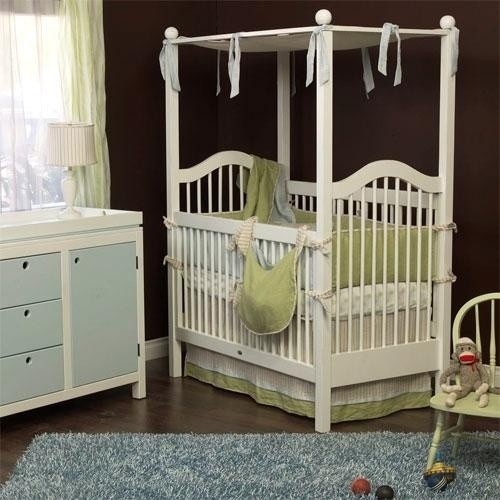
[0,206,147,419]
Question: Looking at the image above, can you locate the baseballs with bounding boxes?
[375,485,393,500]
[352,480,370,495]
[428,474,447,492]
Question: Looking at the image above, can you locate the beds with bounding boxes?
[159,8,460,435]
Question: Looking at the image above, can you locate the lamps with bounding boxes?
[45,123,97,218]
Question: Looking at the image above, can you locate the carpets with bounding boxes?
[1,430,500,500]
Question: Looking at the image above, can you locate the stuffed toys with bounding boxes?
[440,336,491,408]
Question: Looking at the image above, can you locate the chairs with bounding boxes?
[425,293,500,473]
